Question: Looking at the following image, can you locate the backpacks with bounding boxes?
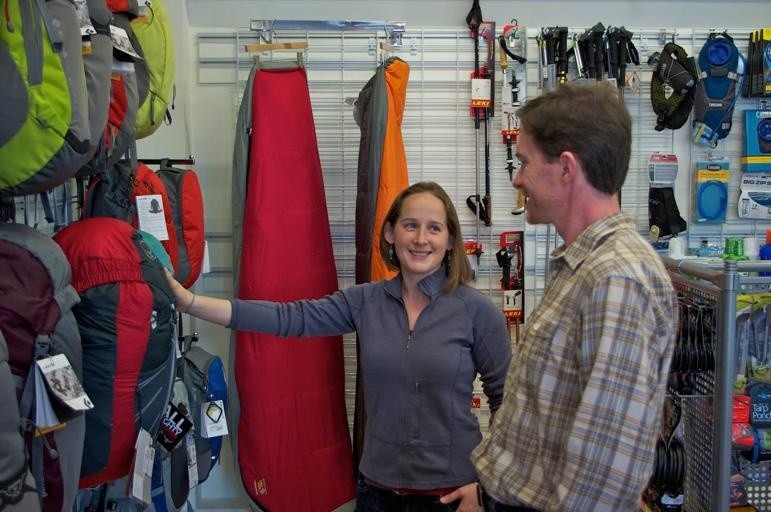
[0,157,228,512]
[1,0,176,194]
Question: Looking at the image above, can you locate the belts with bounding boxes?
[362,475,476,497]
[476,481,542,512]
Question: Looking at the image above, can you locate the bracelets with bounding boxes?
[182,291,197,314]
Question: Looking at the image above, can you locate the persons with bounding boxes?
[471,78,678,512]
[164,181,513,512]
